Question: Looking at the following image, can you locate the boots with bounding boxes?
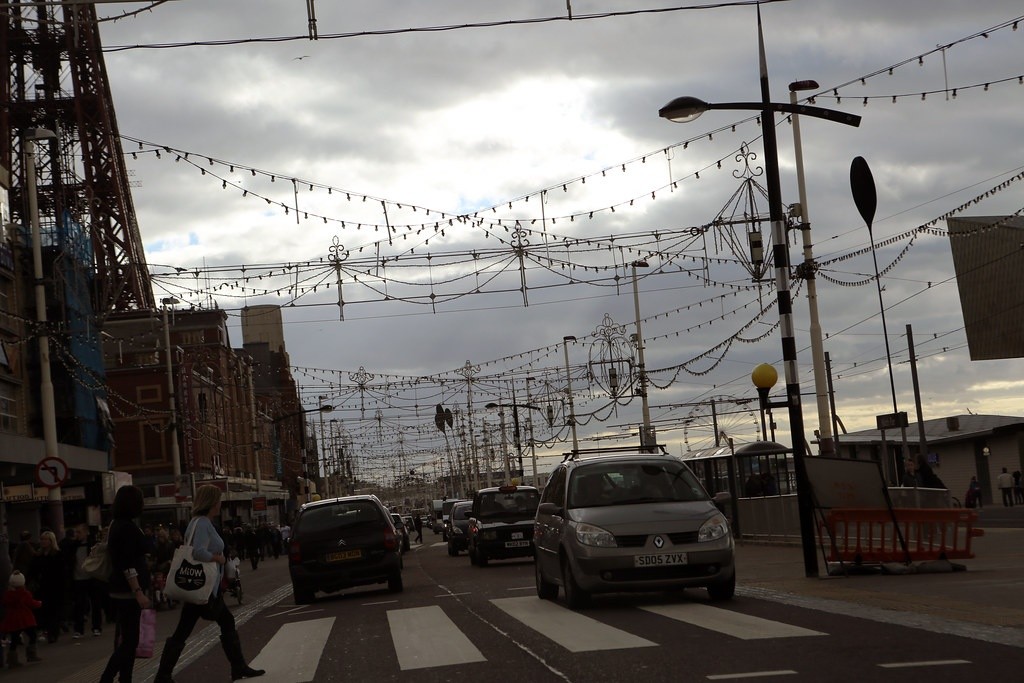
[25,645,43,662]
[153,637,186,683]
[6,651,23,669]
[220,630,266,678]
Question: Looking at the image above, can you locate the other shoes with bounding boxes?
[94,629,101,636]
[72,633,85,639]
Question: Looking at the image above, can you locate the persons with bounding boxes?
[0,524,116,668]
[903,455,933,508]
[216,519,293,605]
[136,520,184,603]
[480,494,507,515]
[745,472,778,497]
[99,485,150,683]
[413,514,424,543]
[997,467,1024,507]
[964,476,982,508]
[393,507,398,513]
[153,485,266,683]
[515,495,526,511]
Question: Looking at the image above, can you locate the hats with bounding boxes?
[9,570,25,587]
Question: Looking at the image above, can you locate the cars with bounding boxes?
[412,499,464,541]
[282,493,404,604]
[464,485,542,565]
[534,444,736,606]
[444,501,472,558]
[401,516,415,532]
[337,507,410,555]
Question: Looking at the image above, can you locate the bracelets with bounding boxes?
[132,587,142,593]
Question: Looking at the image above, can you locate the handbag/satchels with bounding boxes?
[113,608,156,658]
[163,517,218,604]
[79,517,114,586]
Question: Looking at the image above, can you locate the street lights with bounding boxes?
[485,402,525,485]
[563,336,579,458]
[526,376,538,487]
[475,435,480,490]
[25,127,65,538]
[248,361,263,495]
[631,261,650,453]
[162,297,186,519]
[789,79,836,460]
[657,96,820,577]
[300,405,333,501]
[468,444,472,490]
[318,395,331,499]
[330,420,348,497]
[440,457,446,497]
